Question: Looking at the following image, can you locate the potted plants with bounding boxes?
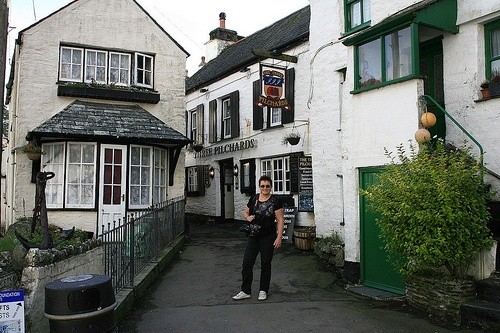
[187,144,203,153]
[480,82,491,98]
[281,133,301,147]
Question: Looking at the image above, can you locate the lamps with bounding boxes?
[233,164,238,177]
[209,167,214,179]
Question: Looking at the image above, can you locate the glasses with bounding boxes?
[260,185,270,188]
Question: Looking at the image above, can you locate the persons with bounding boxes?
[233,176,283,301]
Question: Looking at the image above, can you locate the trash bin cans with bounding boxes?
[44,274,117,333]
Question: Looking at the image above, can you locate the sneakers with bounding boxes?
[231,291,251,300]
[258,290,267,300]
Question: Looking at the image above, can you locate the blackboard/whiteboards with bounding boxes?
[275,207,297,244]
[297,155,314,212]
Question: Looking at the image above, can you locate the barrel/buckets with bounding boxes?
[294,229,316,250]
